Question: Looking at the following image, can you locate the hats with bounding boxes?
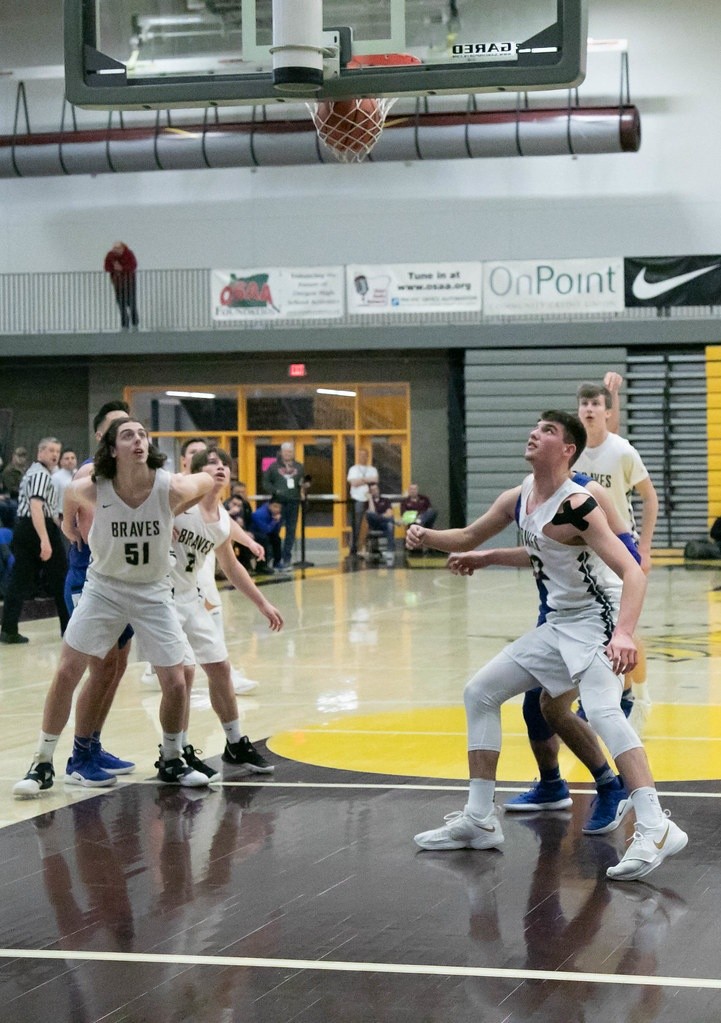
[15,447,29,458]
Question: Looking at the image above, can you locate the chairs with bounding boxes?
[406,508,438,557]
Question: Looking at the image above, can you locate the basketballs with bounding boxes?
[315,95,384,153]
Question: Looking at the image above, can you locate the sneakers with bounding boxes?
[516,811,572,832]
[607,809,688,881]
[65,741,116,786]
[416,847,505,872]
[414,805,505,850]
[13,750,54,799]
[221,735,274,772]
[154,756,209,786]
[90,745,135,774]
[580,774,634,834]
[607,809,687,878]
[608,880,689,931]
[504,777,574,810]
[183,744,220,779]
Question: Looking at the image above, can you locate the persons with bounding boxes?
[104,241,140,332]
[404,409,689,880]
[142,438,265,694]
[63,399,135,786]
[346,448,379,555]
[574,372,658,722]
[11,417,216,796]
[400,483,438,529]
[0,436,70,642]
[169,447,284,781]
[50,450,78,515]
[501,470,634,834]
[224,482,284,574]
[364,482,396,552]
[262,440,304,571]
[0,447,28,498]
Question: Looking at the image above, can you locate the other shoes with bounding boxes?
[273,561,293,571]
[256,563,275,574]
[1,628,29,643]
